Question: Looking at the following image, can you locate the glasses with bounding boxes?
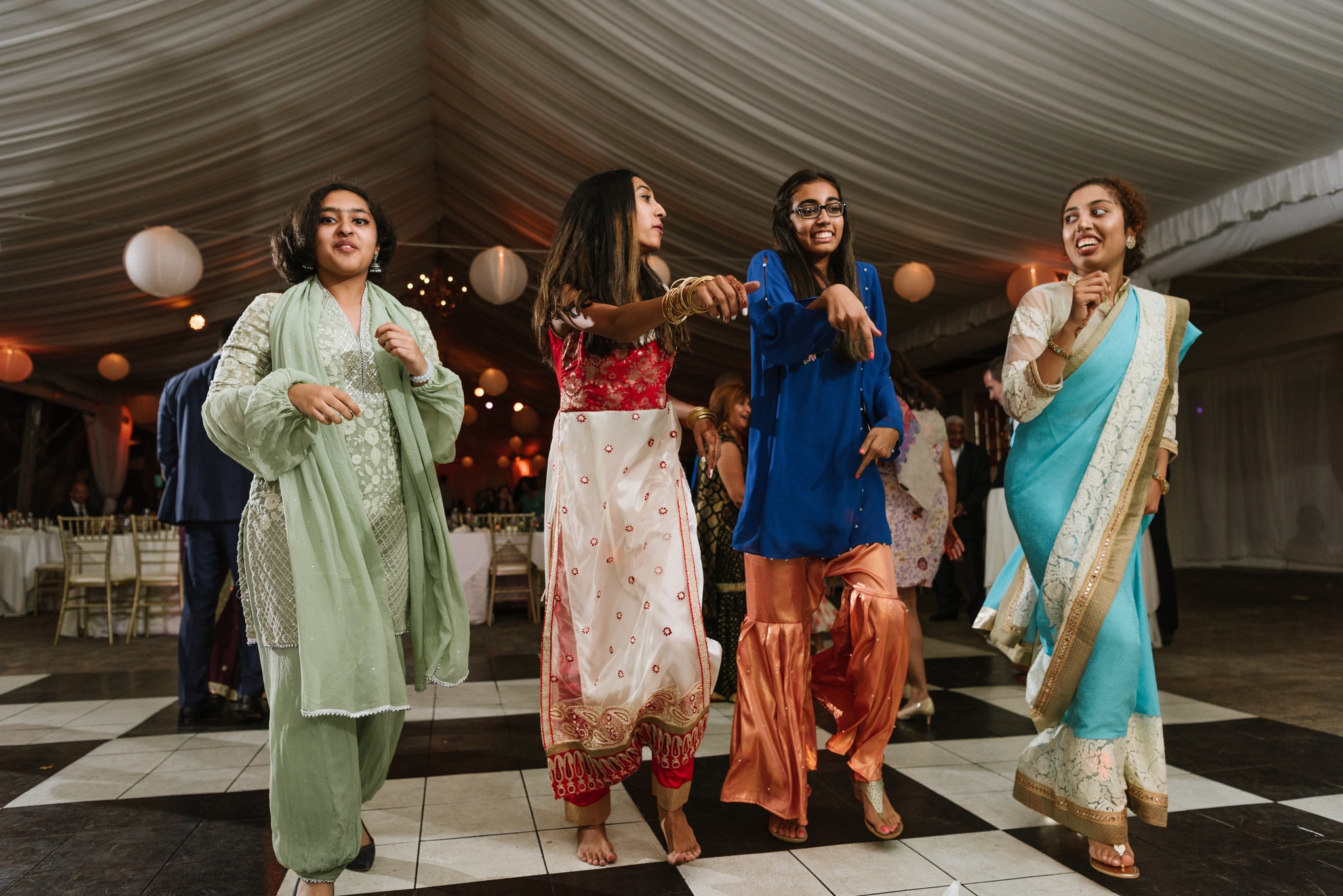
[789,201,846,219]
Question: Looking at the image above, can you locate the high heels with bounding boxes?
[896,698,935,725]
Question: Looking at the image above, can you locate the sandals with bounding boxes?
[850,771,903,839]
[1089,844,1140,879]
[769,809,809,842]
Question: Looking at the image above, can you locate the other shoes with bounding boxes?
[709,689,737,703]
[928,610,981,625]
[345,815,375,872]
[175,696,267,732]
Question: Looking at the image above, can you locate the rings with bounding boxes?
[839,327,849,334]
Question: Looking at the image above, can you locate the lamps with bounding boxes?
[0,349,32,383]
[1007,264,1059,308]
[469,246,527,305]
[894,262,934,302]
[463,403,546,470]
[99,353,129,382]
[406,264,466,318]
[124,226,202,298]
[480,368,507,395]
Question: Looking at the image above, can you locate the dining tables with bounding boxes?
[0,524,70,616]
[59,533,180,638]
[447,532,544,626]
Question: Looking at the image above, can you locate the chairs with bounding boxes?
[32,518,105,619]
[124,515,185,645]
[53,514,137,646]
[534,566,545,625]
[459,512,497,529]
[485,511,537,625]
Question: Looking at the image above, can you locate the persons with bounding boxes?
[810,589,842,654]
[927,414,995,625]
[874,339,965,726]
[198,183,470,895]
[999,174,1202,878]
[982,354,1015,430]
[527,167,760,866]
[720,164,910,843]
[698,381,751,704]
[1145,458,1181,649]
[436,464,549,530]
[41,452,171,537]
[158,331,271,726]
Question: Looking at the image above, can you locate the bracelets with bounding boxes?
[1047,337,1073,361]
[1151,471,1171,495]
[407,357,434,384]
[660,274,715,325]
